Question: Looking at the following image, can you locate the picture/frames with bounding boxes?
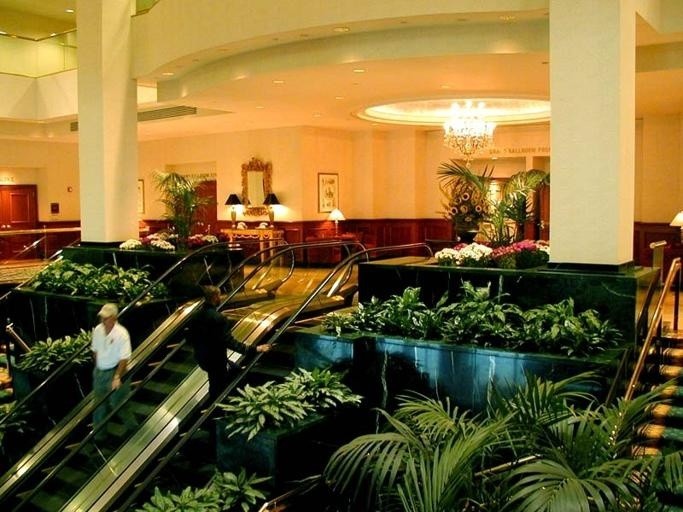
[138,179,145,214]
[318,173,339,213]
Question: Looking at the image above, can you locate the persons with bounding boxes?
[185,285,274,405]
[90,303,139,455]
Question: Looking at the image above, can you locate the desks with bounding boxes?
[220,229,286,267]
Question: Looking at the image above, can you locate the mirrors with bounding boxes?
[241,157,272,216]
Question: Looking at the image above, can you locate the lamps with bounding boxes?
[263,194,280,228]
[224,194,242,228]
[327,209,346,241]
[442,102,497,167]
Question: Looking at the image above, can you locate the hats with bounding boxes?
[97,302,119,318]
